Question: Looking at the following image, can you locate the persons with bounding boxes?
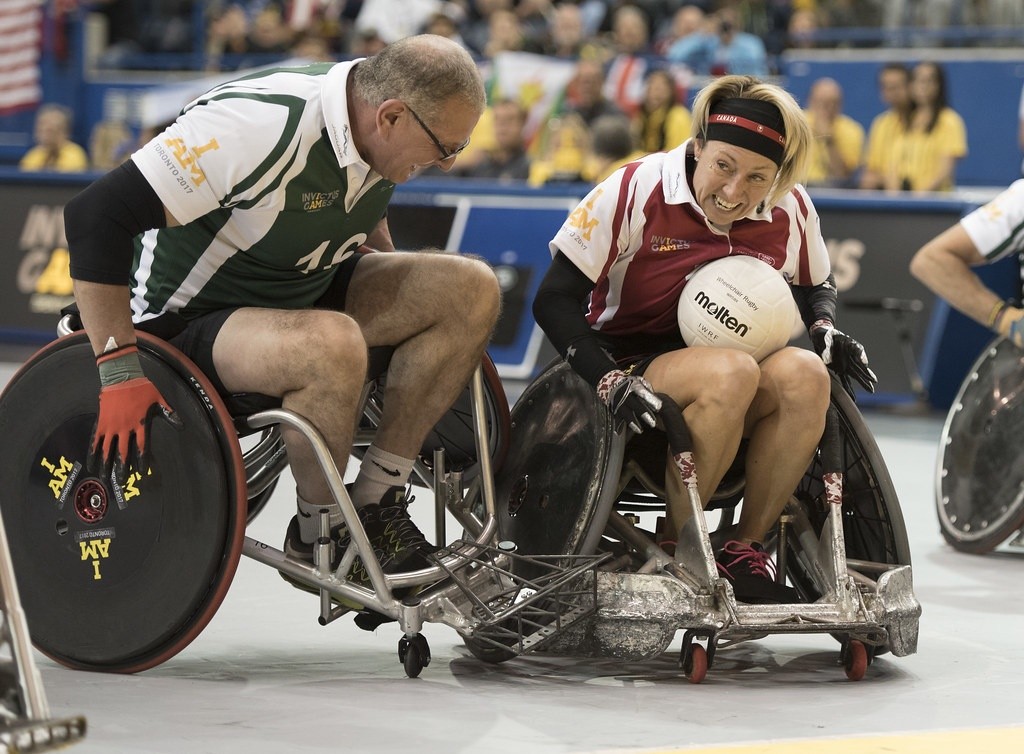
[910,179,1024,347]
[63,34,502,598]
[797,59,967,191]
[1018,78,1024,177]
[532,74,878,603]
[20,0,764,184]
[772,0,1024,49]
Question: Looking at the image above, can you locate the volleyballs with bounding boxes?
[678,255,795,364]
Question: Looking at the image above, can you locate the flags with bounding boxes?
[0,0,43,111]
[602,53,694,118]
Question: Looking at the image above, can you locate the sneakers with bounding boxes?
[277,480,475,618]
[715,536,799,603]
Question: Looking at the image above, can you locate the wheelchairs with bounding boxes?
[933,329,1024,556]
[456,353,923,683]
[0,512,88,754]
[0,246,614,679]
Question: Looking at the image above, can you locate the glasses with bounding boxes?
[402,99,472,160]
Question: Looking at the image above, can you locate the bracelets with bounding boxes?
[989,301,1009,332]
[818,135,835,146]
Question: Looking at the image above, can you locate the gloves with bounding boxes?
[810,317,878,402]
[87,342,186,486]
[604,378,663,433]
